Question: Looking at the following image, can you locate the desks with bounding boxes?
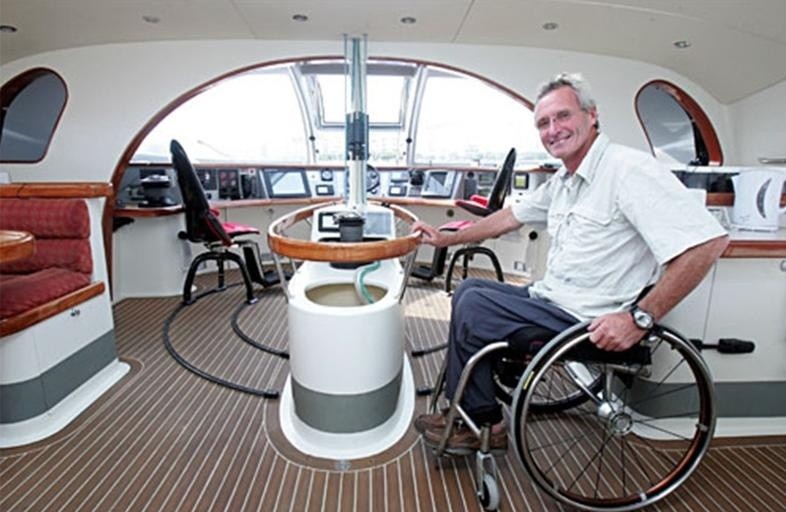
[0,229,37,264]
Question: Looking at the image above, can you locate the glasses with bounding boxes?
[535,106,586,131]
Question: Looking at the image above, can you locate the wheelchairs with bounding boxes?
[412,324,755,506]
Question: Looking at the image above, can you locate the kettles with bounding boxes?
[730,165,786,231]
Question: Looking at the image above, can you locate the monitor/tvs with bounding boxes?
[262,167,312,198]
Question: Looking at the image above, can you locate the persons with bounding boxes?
[412,71,732,459]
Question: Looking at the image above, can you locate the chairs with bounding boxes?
[0,182,130,450]
[410,148,517,292]
[170,139,296,305]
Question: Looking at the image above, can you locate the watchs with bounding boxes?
[628,303,656,332]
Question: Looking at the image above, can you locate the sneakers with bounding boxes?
[423,422,508,457]
[414,408,469,434]
